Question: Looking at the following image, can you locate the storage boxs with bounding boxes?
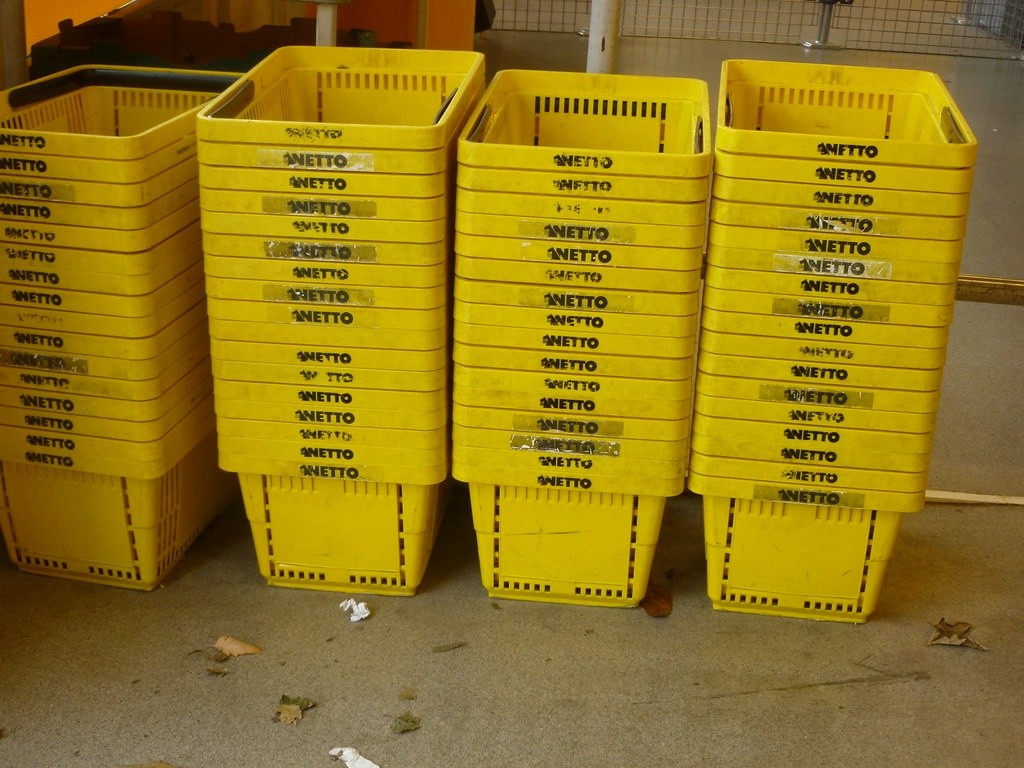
[0,39,983,629]
